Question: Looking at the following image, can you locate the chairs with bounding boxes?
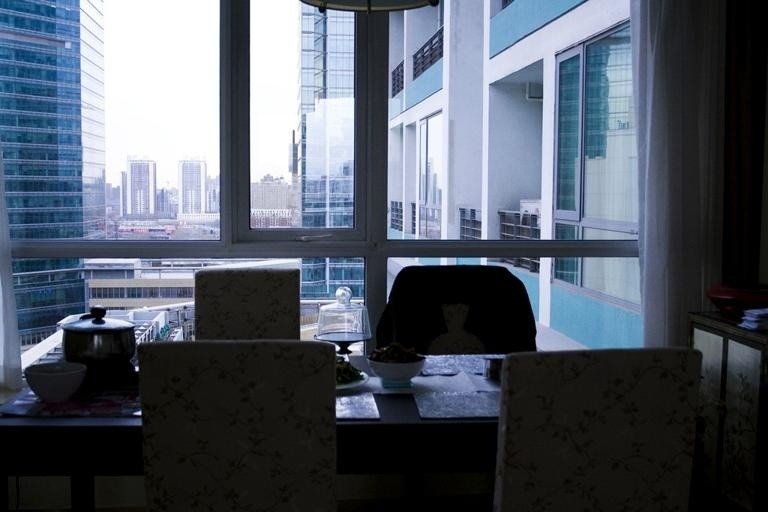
[194,271,301,344]
[377,267,536,352]
[139,341,337,510]
[494,348,702,512]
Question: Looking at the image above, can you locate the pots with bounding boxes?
[61,305,136,365]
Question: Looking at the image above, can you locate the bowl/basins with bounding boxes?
[24,362,86,403]
[365,356,426,385]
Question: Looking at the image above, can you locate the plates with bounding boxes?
[335,370,369,392]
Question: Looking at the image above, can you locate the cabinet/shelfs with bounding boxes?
[686,309,767,491]
